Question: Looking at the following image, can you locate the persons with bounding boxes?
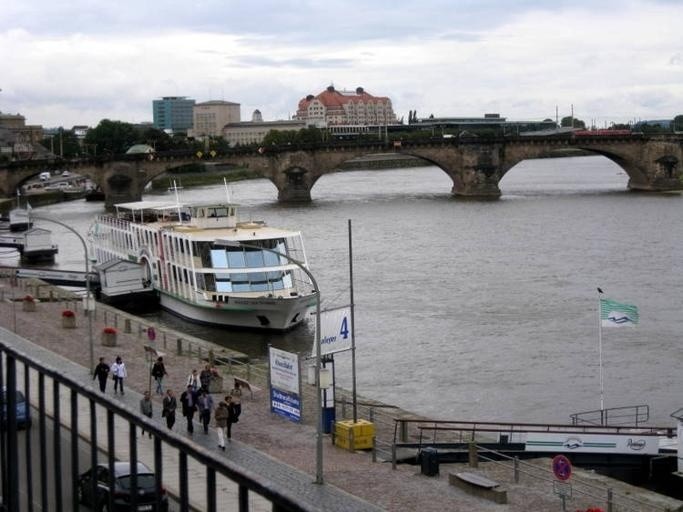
[110,356,127,396]
[161,389,178,430]
[140,391,153,439]
[93,357,110,394]
[151,357,168,399]
[180,365,242,451]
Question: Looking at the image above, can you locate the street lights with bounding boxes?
[207,234,329,487]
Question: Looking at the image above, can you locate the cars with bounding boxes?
[62,171,71,177]
[78,460,171,511]
[0,385,30,430]
[45,182,74,191]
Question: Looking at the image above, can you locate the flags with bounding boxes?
[599,298,640,329]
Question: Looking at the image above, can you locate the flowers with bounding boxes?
[103,327,116,334]
[61,310,74,318]
[23,294,33,302]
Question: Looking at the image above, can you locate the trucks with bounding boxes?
[38,172,51,181]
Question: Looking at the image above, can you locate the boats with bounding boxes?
[85,171,326,331]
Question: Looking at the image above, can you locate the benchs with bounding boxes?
[143,346,167,364]
[445,470,509,506]
[233,377,261,400]
